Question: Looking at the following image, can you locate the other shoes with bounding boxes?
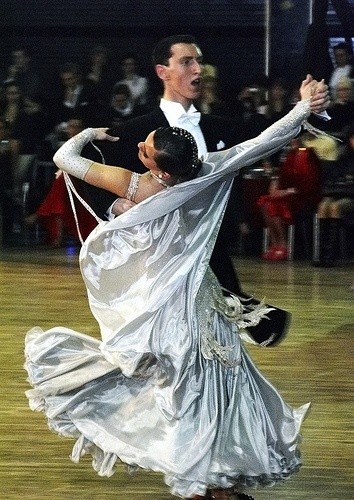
[48,237,62,248]
[185,491,254,500]
[271,246,288,261]
[261,244,278,258]
[22,214,38,224]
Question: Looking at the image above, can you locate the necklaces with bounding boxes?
[149,170,170,188]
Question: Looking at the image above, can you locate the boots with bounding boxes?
[311,217,328,268]
[322,218,342,267]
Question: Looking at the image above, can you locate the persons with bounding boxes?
[0,41,354,167]
[23,75,327,500]
[84,35,330,347]
[24,117,90,247]
[314,129,354,267]
[0,118,19,246]
[258,135,316,260]
[228,173,252,241]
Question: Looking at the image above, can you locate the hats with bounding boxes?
[334,76,352,91]
